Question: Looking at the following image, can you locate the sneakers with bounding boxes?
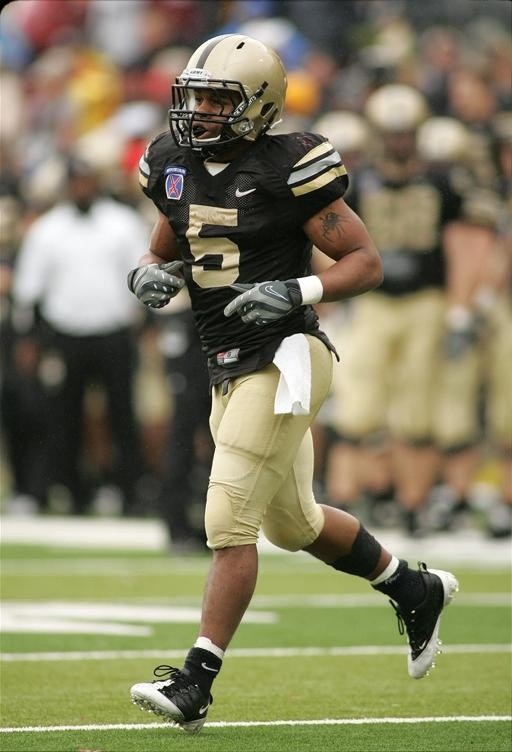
[130,666,210,732]
[388,560,458,679]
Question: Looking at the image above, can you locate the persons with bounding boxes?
[0,3,512,555]
[126,33,457,732]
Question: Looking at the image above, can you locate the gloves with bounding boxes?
[223,279,306,326]
[127,258,183,310]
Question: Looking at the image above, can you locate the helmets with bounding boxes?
[168,33,286,146]
